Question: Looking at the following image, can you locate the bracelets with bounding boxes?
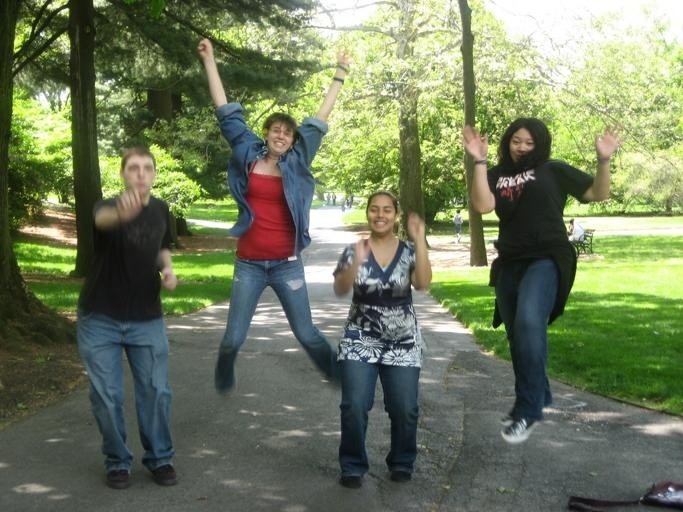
[472,160,488,164]
[335,63,349,73]
[332,77,344,82]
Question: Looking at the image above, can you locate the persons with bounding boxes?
[567,218,585,241]
[197,37,350,388]
[333,191,431,488]
[461,118,623,443]
[327,192,353,211]
[453,210,463,241]
[76,146,177,489]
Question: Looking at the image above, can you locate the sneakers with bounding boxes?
[339,475,362,488]
[498,414,539,445]
[151,465,177,485]
[106,469,131,489]
[390,471,410,483]
